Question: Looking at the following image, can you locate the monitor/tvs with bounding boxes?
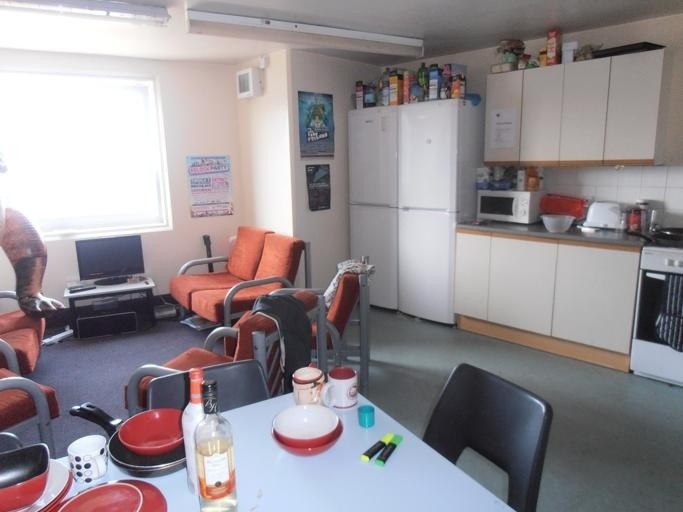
[75,233,146,284]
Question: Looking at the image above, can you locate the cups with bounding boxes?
[291,363,358,410]
[358,405,375,428]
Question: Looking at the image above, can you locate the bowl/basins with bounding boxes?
[271,404,344,457]
[540,214,576,233]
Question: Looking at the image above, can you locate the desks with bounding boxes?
[64,277,156,337]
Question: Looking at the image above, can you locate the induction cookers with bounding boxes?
[643,239,683,250]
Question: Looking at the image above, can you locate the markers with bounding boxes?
[374,435,403,466]
[360,431,394,461]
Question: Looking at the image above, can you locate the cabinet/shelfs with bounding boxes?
[488,227,642,373]
[562,48,683,166]
[484,64,563,166]
[454,222,492,341]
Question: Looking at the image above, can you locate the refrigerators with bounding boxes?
[346,98,485,327]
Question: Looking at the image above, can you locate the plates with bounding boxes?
[7,458,168,512]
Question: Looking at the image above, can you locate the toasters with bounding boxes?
[583,201,622,229]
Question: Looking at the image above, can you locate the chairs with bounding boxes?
[144,361,269,414]
[124,288,325,420]
[424,362,552,511]
[264,255,370,396]
[0,338,60,457]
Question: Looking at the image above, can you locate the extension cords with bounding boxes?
[42,329,72,346]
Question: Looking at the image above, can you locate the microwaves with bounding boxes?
[476,188,548,225]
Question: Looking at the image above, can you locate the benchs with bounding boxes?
[170,226,310,326]
[1,290,72,377]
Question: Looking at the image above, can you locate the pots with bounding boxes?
[644,225,683,246]
[0,400,186,512]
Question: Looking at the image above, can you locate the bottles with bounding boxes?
[355,61,466,109]
[618,201,658,235]
[179,367,238,512]
[517,28,562,69]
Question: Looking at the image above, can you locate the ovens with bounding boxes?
[630,247,683,388]
